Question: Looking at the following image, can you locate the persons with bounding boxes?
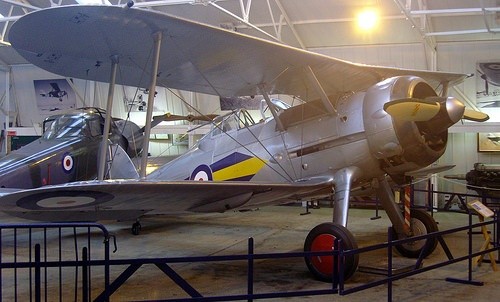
[466,163,500,196]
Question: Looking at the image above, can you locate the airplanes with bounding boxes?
[0,106,171,188]
[39,83,68,102]
[0,1,475,284]
